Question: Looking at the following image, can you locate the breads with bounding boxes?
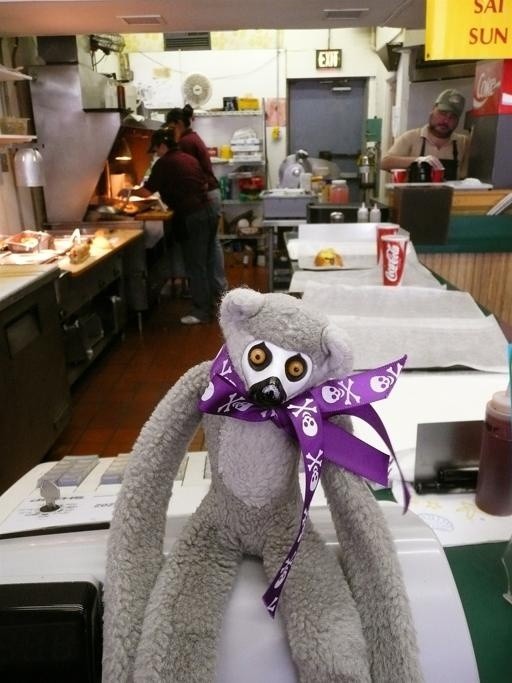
[315,251,343,267]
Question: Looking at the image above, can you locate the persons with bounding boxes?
[375,85,471,178]
[118,128,219,327]
[160,104,231,303]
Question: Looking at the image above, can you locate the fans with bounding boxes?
[181,73,212,114]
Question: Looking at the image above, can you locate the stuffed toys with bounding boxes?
[91,273,427,676]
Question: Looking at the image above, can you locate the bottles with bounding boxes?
[356,202,369,224]
[369,203,382,222]
[219,176,233,201]
[474,381,512,517]
[328,179,350,204]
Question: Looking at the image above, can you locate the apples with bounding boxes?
[68,243,90,263]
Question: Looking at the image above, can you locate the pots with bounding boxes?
[93,201,159,215]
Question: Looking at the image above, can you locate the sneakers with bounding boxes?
[180,314,203,325]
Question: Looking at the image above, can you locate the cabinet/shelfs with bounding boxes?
[196,110,269,241]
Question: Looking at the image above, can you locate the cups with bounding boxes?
[376,224,400,269]
[379,235,410,287]
[431,168,445,183]
[391,168,407,182]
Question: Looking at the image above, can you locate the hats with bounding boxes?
[147,130,174,152]
[435,89,465,117]
[161,108,187,128]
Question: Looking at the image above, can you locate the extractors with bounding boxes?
[26,33,168,221]
